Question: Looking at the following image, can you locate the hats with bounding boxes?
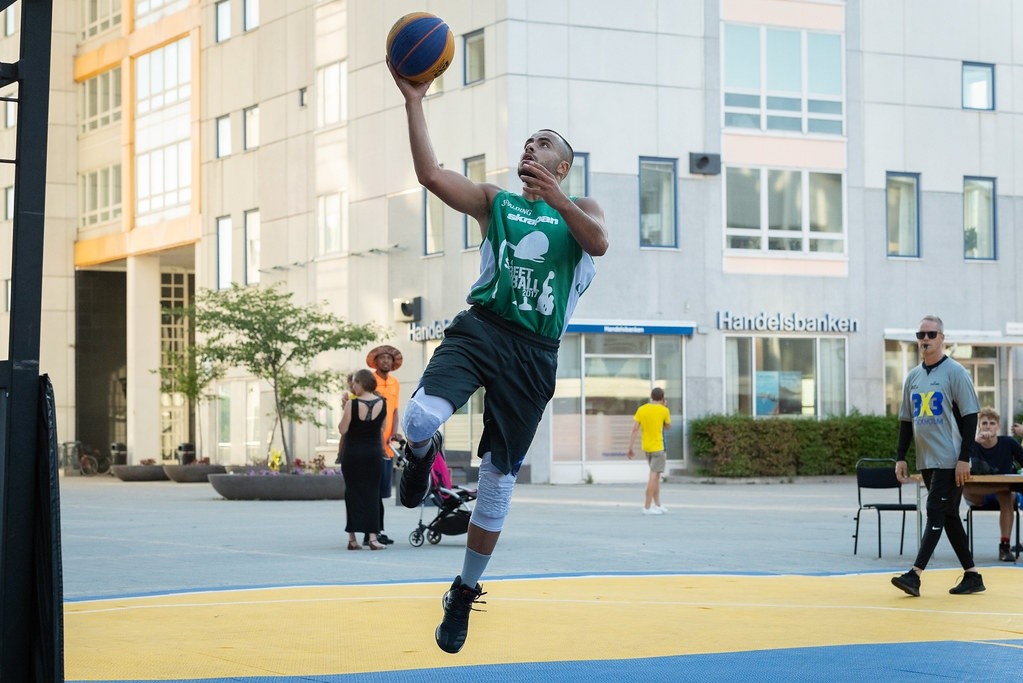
[366,345,403,371]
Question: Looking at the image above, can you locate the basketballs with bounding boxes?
[386,12,455,82]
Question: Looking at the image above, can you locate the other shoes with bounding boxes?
[364,533,393,546]
[369,539,387,549]
[348,539,364,551]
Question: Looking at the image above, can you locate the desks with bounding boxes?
[909,474,1023,561]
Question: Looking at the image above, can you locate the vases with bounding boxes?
[162,464,268,483]
[207,474,347,501]
[109,464,171,481]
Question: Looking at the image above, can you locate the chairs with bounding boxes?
[851,458,1023,561]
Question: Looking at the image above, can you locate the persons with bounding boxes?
[891,316,985,597]
[963,407,1023,561]
[366,345,403,499]
[338,370,394,550]
[627,388,671,514]
[385,54,608,652]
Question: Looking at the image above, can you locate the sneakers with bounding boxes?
[891,570,920,597]
[435,575,482,654]
[400,431,442,508]
[655,505,669,512]
[642,507,662,515]
[999,542,1016,561]
[950,572,986,595]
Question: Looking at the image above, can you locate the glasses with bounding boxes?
[916,331,943,340]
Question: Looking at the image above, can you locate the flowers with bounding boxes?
[227,451,342,475]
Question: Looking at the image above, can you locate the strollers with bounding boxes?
[386,436,477,548]
